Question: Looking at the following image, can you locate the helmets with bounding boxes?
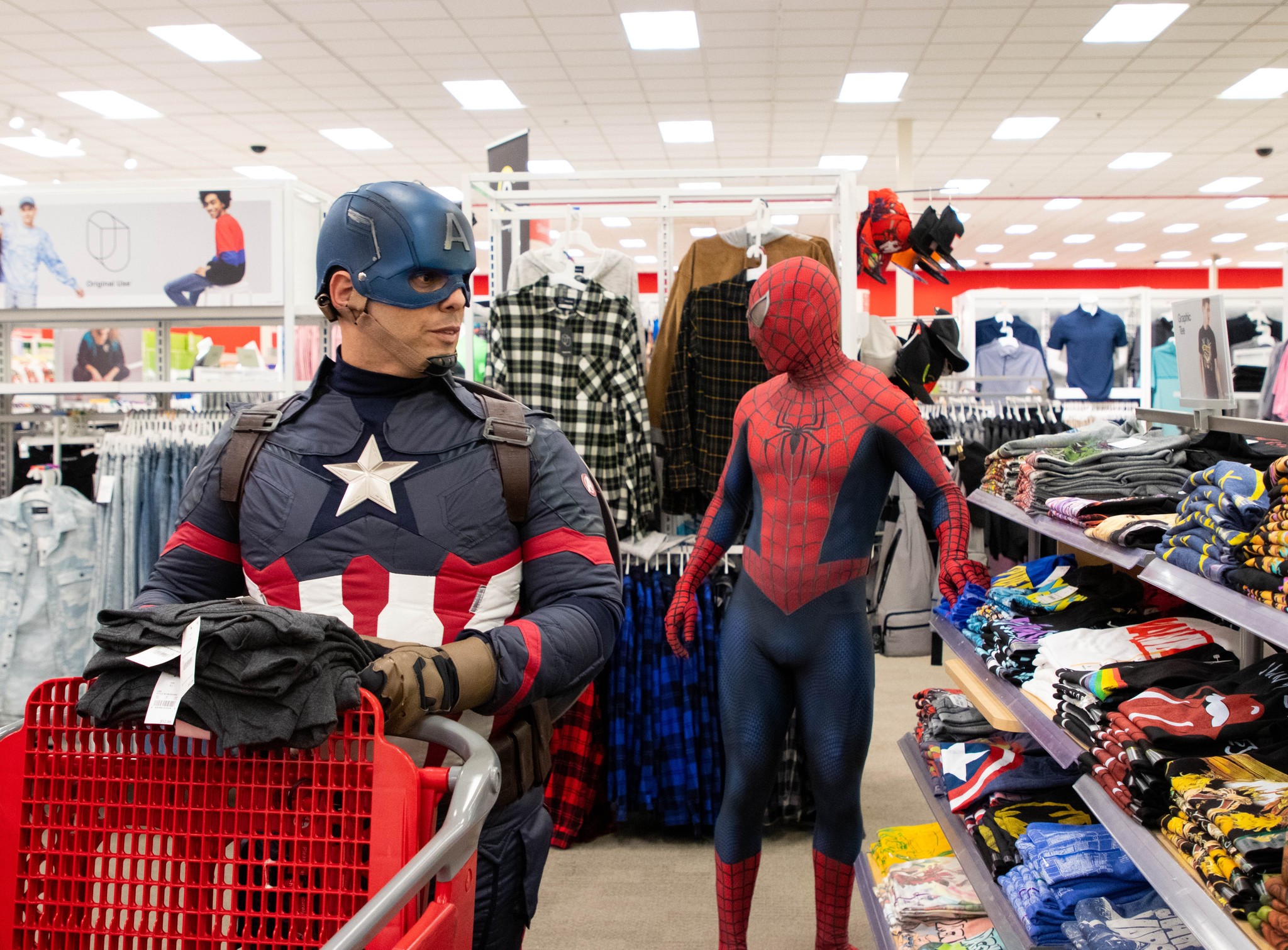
[313,180,476,312]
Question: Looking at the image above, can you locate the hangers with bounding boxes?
[745,197,770,281]
[995,302,1019,348]
[916,395,1057,424]
[548,209,599,290]
[18,408,233,521]
[624,535,728,576]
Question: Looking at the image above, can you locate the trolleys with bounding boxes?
[0,675,503,950]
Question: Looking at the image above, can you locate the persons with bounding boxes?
[162,189,247,306]
[137,181,623,950]
[665,256,994,950]
[72,327,130,381]
[0,194,87,312]
[1196,297,1223,400]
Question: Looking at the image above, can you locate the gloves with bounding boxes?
[354,633,498,738]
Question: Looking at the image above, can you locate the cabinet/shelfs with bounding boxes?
[851,487,1288,950]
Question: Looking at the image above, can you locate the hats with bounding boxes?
[859,305,970,404]
[857,186,966,286]
[955,436,992,528]
